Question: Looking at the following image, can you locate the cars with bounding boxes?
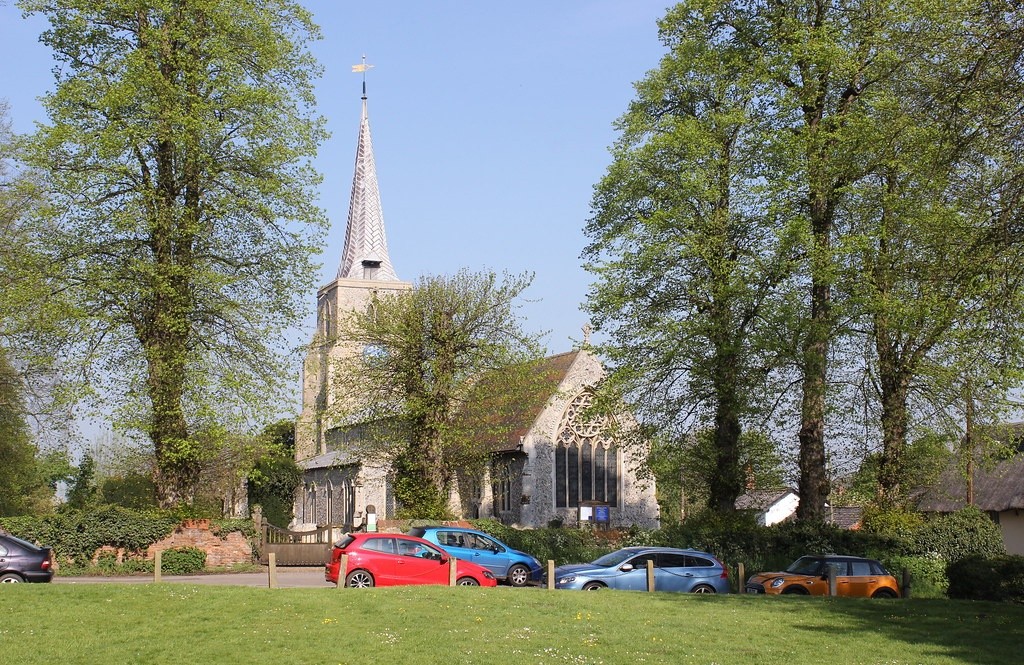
[543,546,732,594]
[745,556,900,598]
[324,532,495,587]
[0,531,56,584]
[401,525,545,586]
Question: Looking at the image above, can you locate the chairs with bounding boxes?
[459,536,464,547]
